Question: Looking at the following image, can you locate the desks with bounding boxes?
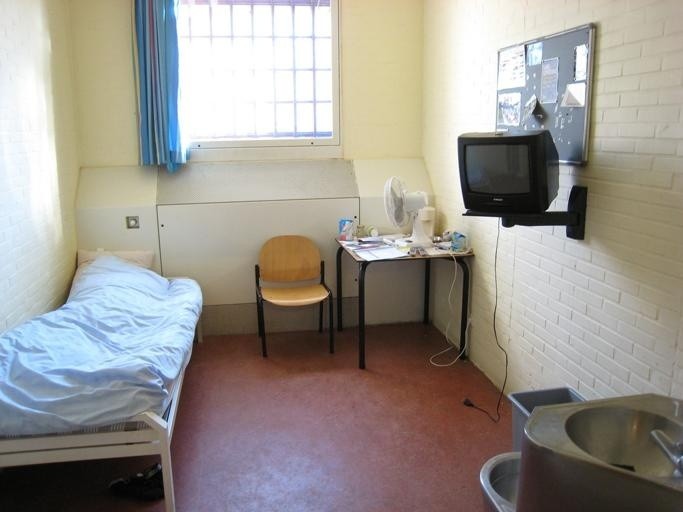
[335,233,475,370]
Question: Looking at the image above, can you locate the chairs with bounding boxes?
[255,235,335,358]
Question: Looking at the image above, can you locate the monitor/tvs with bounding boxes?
[457,129,560,212]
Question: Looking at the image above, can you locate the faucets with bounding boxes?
[647,428,683,476]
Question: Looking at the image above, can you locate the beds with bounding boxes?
[0,249,203,512]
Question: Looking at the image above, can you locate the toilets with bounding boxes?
[480,451,521,512]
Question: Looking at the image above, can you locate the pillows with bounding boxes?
[77,249,155,270]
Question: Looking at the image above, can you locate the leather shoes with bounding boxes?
[112,462,165,501]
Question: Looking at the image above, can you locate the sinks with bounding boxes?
[564,405,682,480]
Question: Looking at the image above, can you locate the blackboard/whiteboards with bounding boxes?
[494,22,597,167]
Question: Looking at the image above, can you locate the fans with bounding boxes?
[383,176,436,248]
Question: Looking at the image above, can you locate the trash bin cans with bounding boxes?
[505,386,589,453]
